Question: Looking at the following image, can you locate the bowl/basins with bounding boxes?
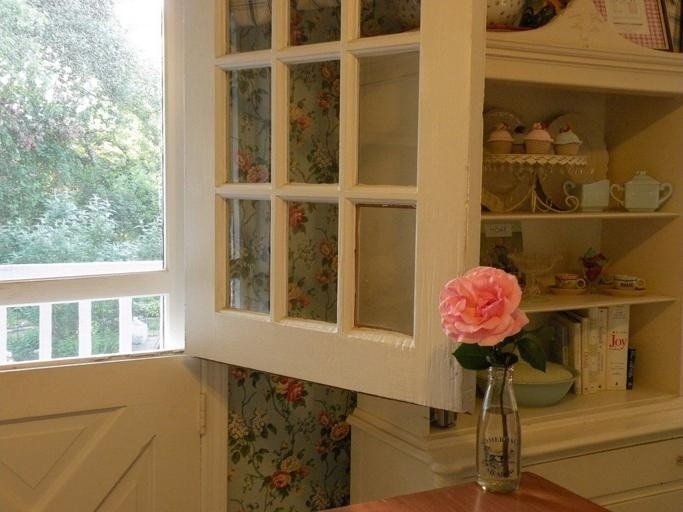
[478,357,578,406]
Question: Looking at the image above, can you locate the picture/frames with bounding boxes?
[594,1,674,52]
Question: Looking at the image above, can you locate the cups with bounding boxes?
[584,260,613,292]
[553,273,585,290]
[610,274,645,290]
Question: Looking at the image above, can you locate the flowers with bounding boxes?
[437,266,546,474]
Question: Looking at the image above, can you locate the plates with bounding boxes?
[483,108,609,213]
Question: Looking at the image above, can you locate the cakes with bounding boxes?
[487,122,514,154]
[509,126,526,154]
[523,122,554,154]
[553,123,583,156]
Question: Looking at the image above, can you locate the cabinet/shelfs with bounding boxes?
[346,1,683,512]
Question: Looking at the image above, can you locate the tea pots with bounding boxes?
[609,171,674,213]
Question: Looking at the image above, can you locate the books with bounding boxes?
[546,305,632,397]
